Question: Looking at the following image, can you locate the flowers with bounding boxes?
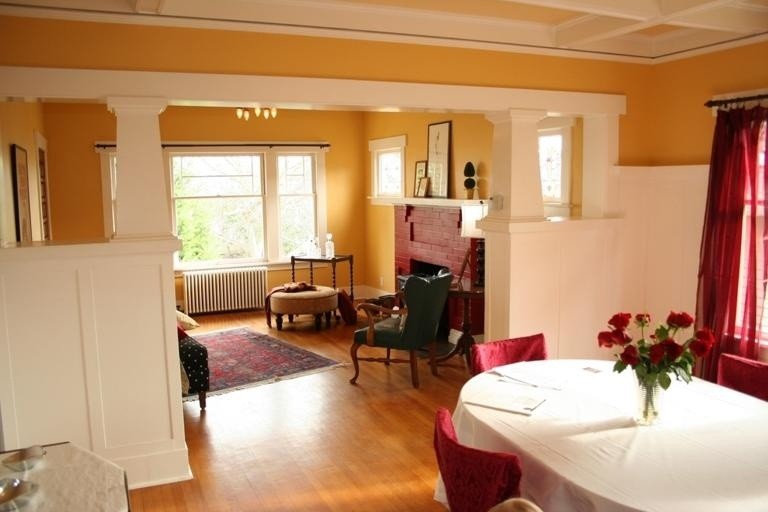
[597,311,715,421]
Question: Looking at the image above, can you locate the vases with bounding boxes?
[632,373,662,425]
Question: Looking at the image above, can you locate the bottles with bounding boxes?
[325,231,334,259]
[307,239,321,258]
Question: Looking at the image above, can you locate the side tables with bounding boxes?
[427,277,484,375]
[291,254,354,318]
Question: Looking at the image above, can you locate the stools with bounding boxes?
[178,336,208,410]
[270,284,338,331]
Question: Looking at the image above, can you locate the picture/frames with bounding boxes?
[426,121,451,198]
[414,160,427,198]
[417,177,430,198]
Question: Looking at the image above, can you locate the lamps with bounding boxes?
[235,108,277,121]
[461,204,488,287]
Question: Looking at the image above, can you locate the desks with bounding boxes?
[433,358,768,512]
[0,441,131,512]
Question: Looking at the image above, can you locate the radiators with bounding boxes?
[183,266,268,318]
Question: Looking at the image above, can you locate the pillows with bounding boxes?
[176,309,200,330]
[177,321,189,337]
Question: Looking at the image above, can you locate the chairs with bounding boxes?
[472,332,547,378]
[718,353,768,403]
[350,273,452,388]
[433,407,522,512]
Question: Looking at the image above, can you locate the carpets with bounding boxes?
[180,326,348,402]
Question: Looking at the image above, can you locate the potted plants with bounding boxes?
[464,162,476,198]
